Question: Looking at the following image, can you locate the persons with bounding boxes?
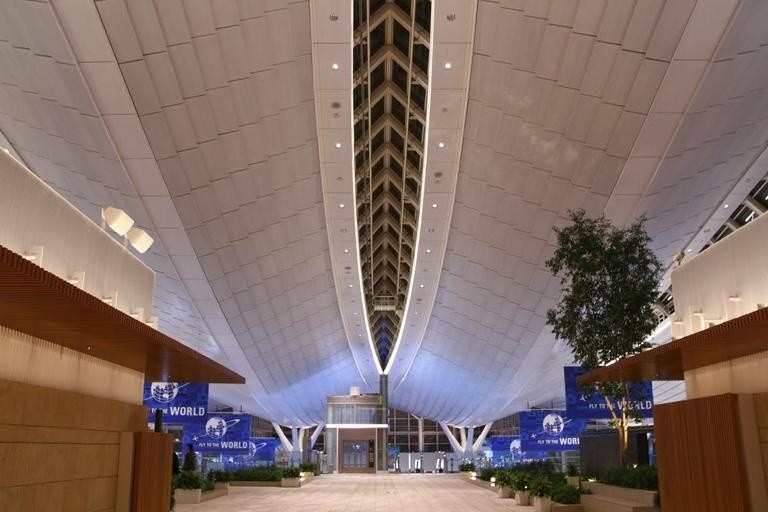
[173,452,181,474]
[181,443,197,476]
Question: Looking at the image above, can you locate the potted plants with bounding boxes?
[300,463,314,477]
[495,459,581,511]
[174,472,201,504]
[280,469,301,486]
[211,471,231,489]
